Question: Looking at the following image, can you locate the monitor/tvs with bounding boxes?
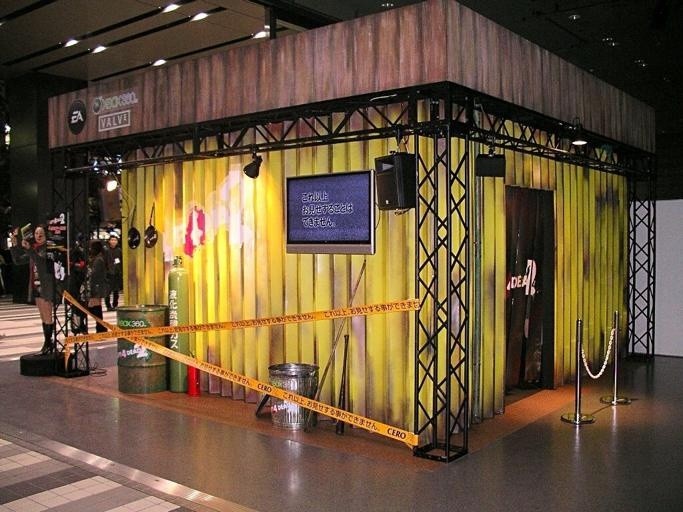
[283,170,377,257]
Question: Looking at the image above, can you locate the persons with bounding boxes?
[68,233,90,332]
[105,235,121,311]
[8,223,60,356]
[87,240,108,332]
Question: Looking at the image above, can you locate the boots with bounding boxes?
[33,321,55,355]
[89,304,108,333]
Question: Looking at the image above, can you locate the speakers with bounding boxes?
[98,186,121,222]
[373,153,416,210]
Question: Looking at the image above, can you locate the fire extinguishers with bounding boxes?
[187,351,199,394]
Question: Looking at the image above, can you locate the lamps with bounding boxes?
[569,127,591,147]
[240,146,263,180]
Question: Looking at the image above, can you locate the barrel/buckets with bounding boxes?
[116,305,168,393]
